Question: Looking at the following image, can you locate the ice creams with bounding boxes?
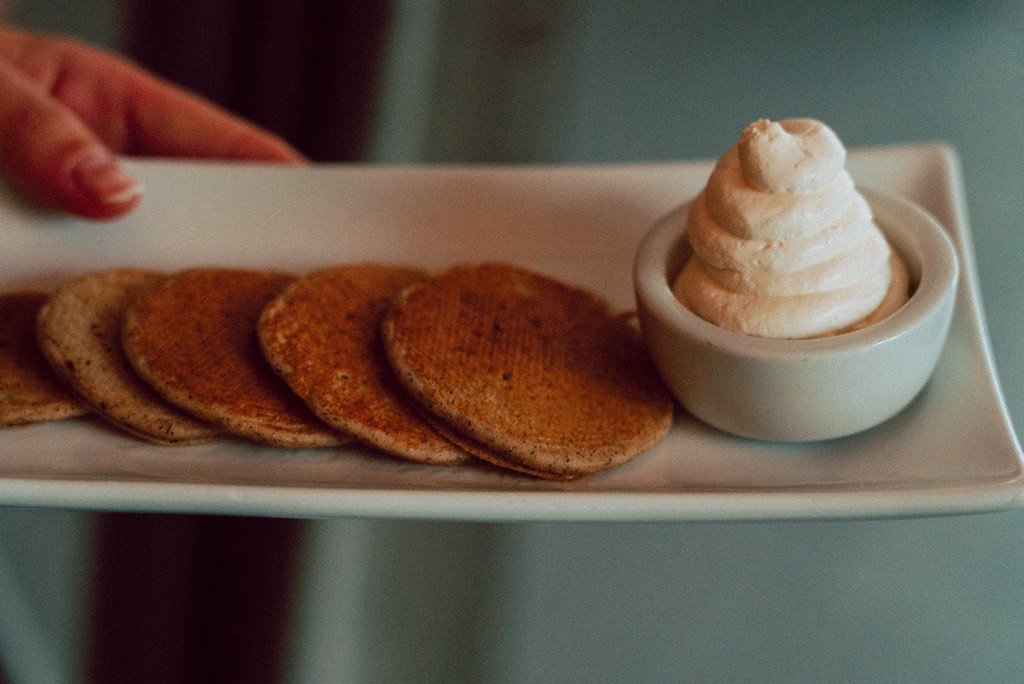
[668,118,912,341]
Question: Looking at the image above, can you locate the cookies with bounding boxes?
[1,261,676,483]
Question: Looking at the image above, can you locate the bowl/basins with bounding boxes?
[632,185,961,444]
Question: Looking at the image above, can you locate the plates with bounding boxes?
[2,140,1024,523]
